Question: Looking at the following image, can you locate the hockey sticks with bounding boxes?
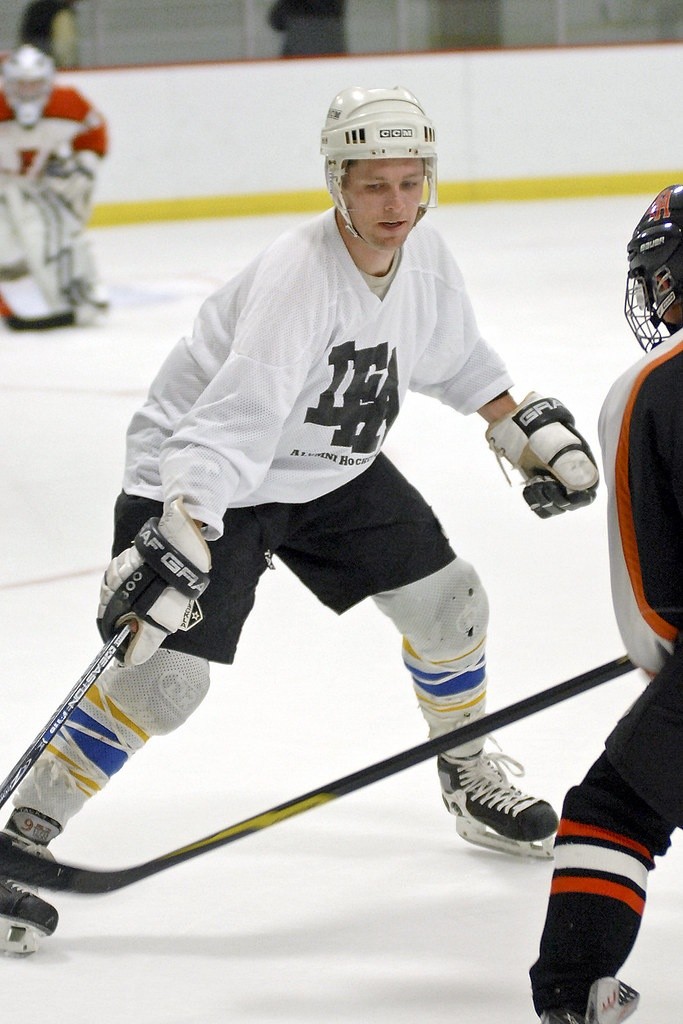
[0,650,638,896]
[0,289,80,336]
[0,615,142,813]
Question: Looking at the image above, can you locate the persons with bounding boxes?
[525,186,682,1023]
[0,89,597,954]
[18,0,78,73]
[268,1,346,59]
[0,43,109,326]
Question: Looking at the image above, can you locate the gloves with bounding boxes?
[486,391,599,520]
[96,495,212,668]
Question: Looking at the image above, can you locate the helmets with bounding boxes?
[1,43,55,130]
[320,85,436,223]
[624,184,683,354]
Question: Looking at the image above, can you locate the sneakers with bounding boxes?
[436,753,560,861]
[0,807,62,955]
[529,961,639,1024]
